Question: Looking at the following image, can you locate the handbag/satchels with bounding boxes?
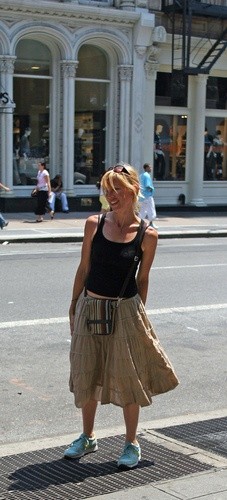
[84,299,114,335]
[31,189,37,197]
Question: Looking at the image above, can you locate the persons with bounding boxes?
[0,182,11,229]
[89,149,93,160]
[48,175,69,215]
[139,164,158,229]
[19,127,31,184]
[31,162,54,223]
[81,156,88,167]
[154,124,165,181]
[98,124,105,176]
[65,163,179,470]
[204,127,214,181]
[213,130,224,179]
[75,127,85,172]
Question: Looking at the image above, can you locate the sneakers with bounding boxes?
[117,443,141,467]
[64,433,98,458]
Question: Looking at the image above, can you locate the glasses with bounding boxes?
[108,165,130,174]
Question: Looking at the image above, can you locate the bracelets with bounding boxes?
[72,299,78,301]
